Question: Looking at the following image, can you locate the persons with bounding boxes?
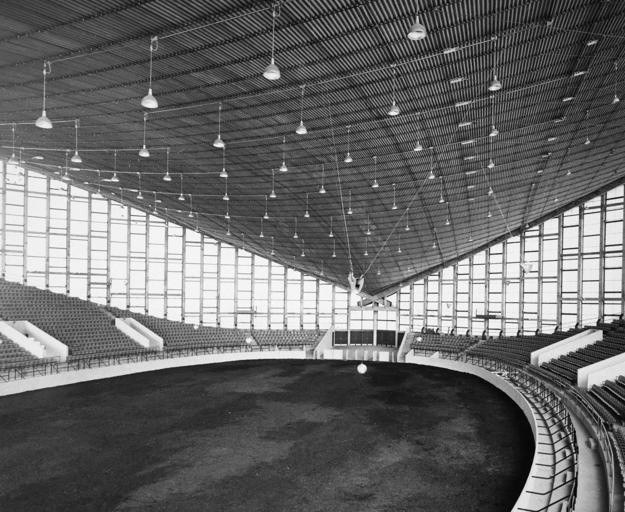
[421,311,624,340]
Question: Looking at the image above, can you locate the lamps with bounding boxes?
[340,40,621,280]
[409,2,427,42]
[36,61,54,129]
[264,6,280,80]
[142,38,159,108]
[6,128,336,277]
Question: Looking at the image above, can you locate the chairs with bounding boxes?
[409,318,625,512]
[0,277,326,371]
